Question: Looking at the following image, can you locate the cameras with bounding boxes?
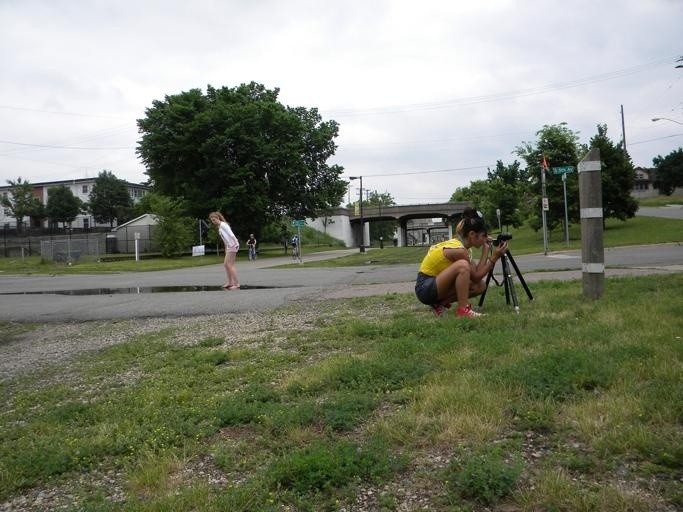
[493,231,513,245]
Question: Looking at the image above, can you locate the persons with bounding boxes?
[245,232,259,262]
[411,206,509,322]
[378,236,384,248]
[208,210,242,291]
[290,234,301,263]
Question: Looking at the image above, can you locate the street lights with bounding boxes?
[350,175,366,253]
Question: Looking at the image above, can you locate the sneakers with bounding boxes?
[431,302,451,319]
[456,304,487,318]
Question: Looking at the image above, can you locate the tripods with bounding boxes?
[478,240,534,316]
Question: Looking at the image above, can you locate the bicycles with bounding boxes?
[292,246,300,260]
[248,244,257,261]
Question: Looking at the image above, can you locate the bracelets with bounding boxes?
[488,257,496,265]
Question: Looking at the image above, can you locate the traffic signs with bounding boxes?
[552,165,576,175]
